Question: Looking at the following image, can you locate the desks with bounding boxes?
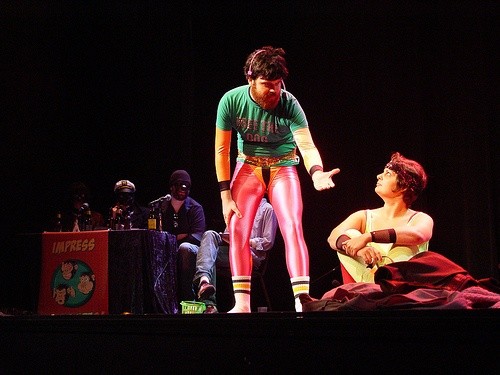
[41,228,177,315]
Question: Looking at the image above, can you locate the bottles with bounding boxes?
[56,201,164,232]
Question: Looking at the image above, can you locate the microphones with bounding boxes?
[281,80,286,100]
[79,202,89,212]
[148,194,171,205]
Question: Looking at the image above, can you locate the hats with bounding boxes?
[169,170,191,186]
[113,179,136,196]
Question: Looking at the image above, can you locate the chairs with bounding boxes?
[216,253,270,311]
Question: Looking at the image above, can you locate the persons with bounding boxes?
[215,46,340,313]
[109,180,151,228]
[327,152,434,290]
[153,170,205,307]
[191,197,277,315]
[56,182,104,230]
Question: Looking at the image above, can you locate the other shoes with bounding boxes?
[204,305,218,314]
[196,280,216,300]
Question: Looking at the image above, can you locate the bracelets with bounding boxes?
[335,234,351,251]
[370,228,397,244]
